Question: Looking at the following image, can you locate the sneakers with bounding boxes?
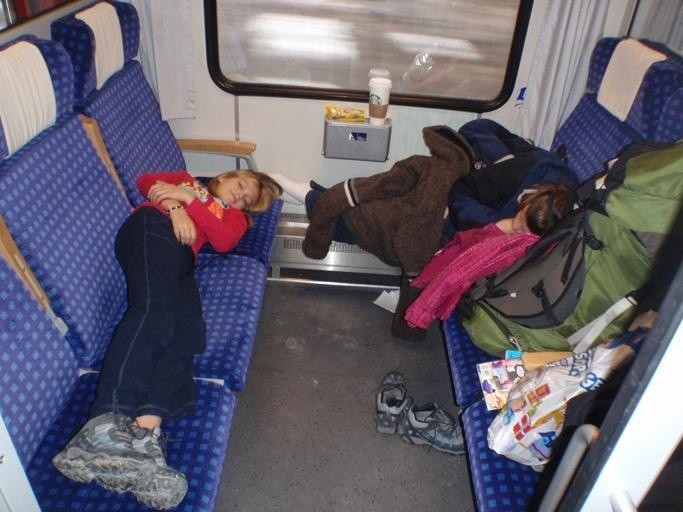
[375,372,408,434]
[131,426,188,510]
[397,400,467,455]
[53,412,156,494]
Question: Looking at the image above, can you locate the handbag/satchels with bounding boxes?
[486,325,650,474]
[459,206,655,357]
[576,135,683,235]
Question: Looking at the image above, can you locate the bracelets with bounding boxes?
[166,204,183,215]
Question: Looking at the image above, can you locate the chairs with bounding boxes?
[0,0,285,512]
[441,37,683,512]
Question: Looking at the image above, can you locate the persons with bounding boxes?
[304,124,584,250]
[49,167,284,512]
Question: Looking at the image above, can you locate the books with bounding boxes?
[473,357,529,412]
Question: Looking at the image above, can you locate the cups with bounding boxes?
[367,78,391,128]
